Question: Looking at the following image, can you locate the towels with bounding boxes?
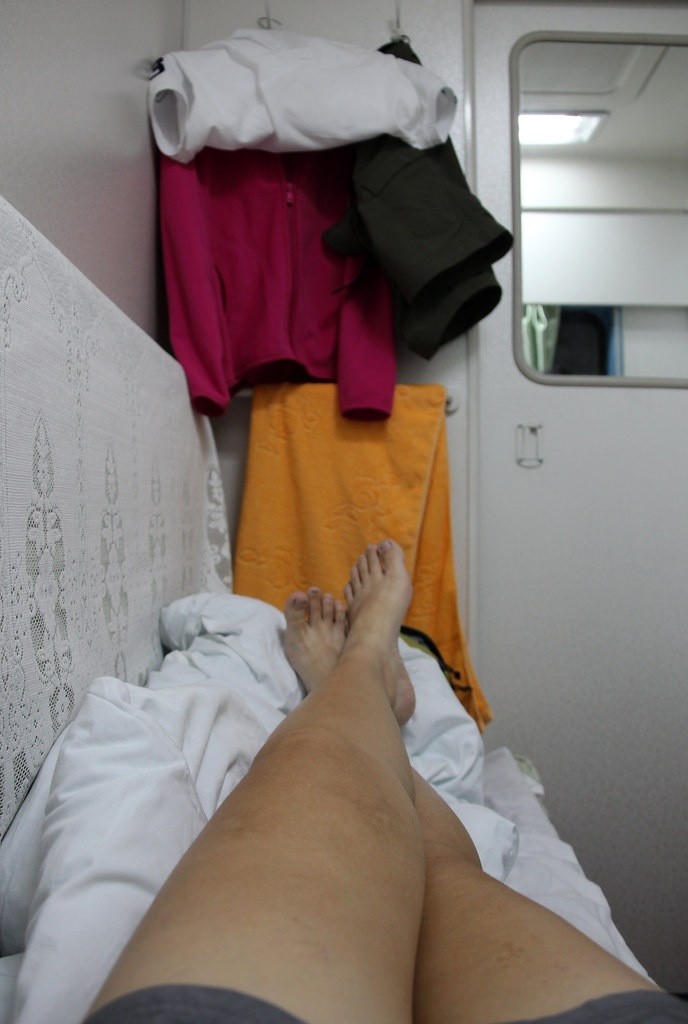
[233,384,494,735]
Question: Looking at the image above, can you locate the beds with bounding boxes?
[2,619,657,1020]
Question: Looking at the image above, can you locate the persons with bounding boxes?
[82,539,687,1023]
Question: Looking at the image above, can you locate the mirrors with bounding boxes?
[510,30,688,389]
